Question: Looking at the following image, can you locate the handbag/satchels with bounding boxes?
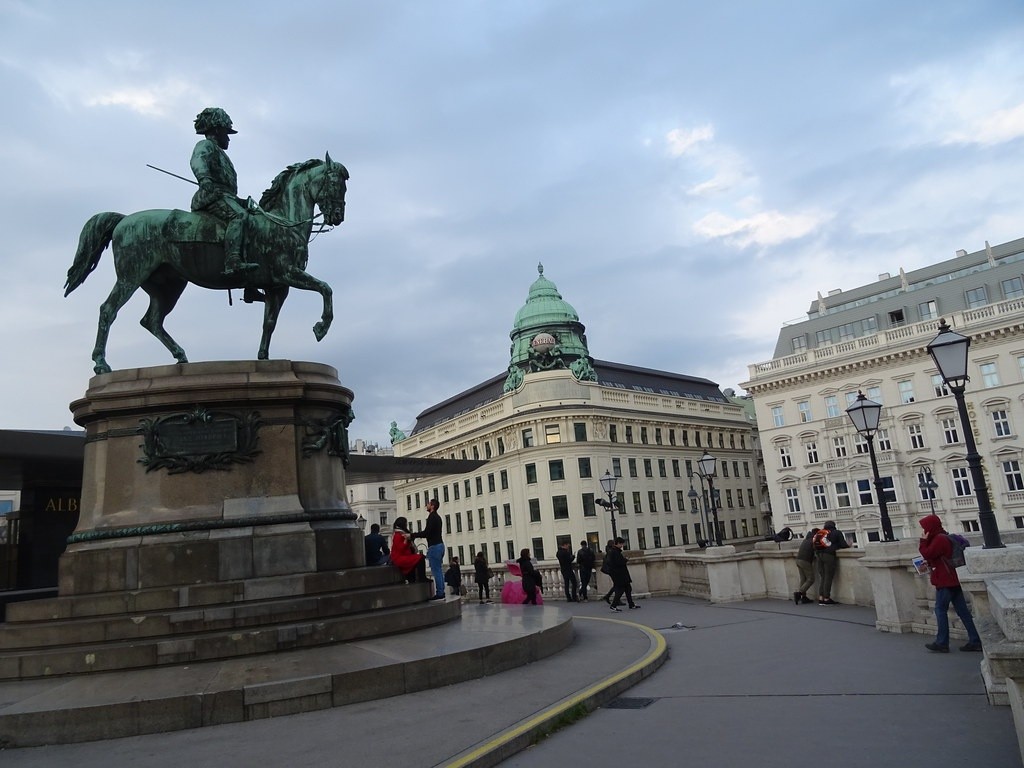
[478,574,488,584]
[601,563,610,575]
[487,568,494,579]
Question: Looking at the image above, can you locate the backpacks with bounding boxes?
[444,570,450,582]
[775,527,793,550]
[945,532,970,568]
[812,529,832,549]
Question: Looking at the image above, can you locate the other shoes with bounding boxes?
[573,596,579,602]
[480,601,485,605]
[486,600,494,604]
[818,599,823,606]
[823,598,839,606]
[422,576,433,582]
[802,596,814,603]
[428,595,446,602]
[578,592,581,601]
[610,606,622,613]
[629,605,641,610]
[617,601,627,605]
[794,592,802,605]
[924,642,949,653]
[959,643,981,651]
[602,596,611,605]
[567,597,575,602]
[583,598,588,602]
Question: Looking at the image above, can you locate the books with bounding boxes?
[913,559,930,575]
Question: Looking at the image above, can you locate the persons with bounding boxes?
[575,352,590,381]
[365,524,391,566]
[389,422,399,444]
[450,556,462,595]
[919,515,982,652]
[815,521,852,606]
[793,528,821,605]
[390,499,446,601]
[503,345,566,393]
[191,107,266,304]
[556,541,578,602]
[577,541,596,602]
[474,552,493,604]
[516,549,543,605]
[603,537,641,611]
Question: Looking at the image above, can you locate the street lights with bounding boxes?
[598,468,618,542]
[844,389,900,543]
[918,464,938,516]
[925,317,1007,549]
[697,448,724,546]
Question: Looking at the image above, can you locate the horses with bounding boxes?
[63,150,350,376]
